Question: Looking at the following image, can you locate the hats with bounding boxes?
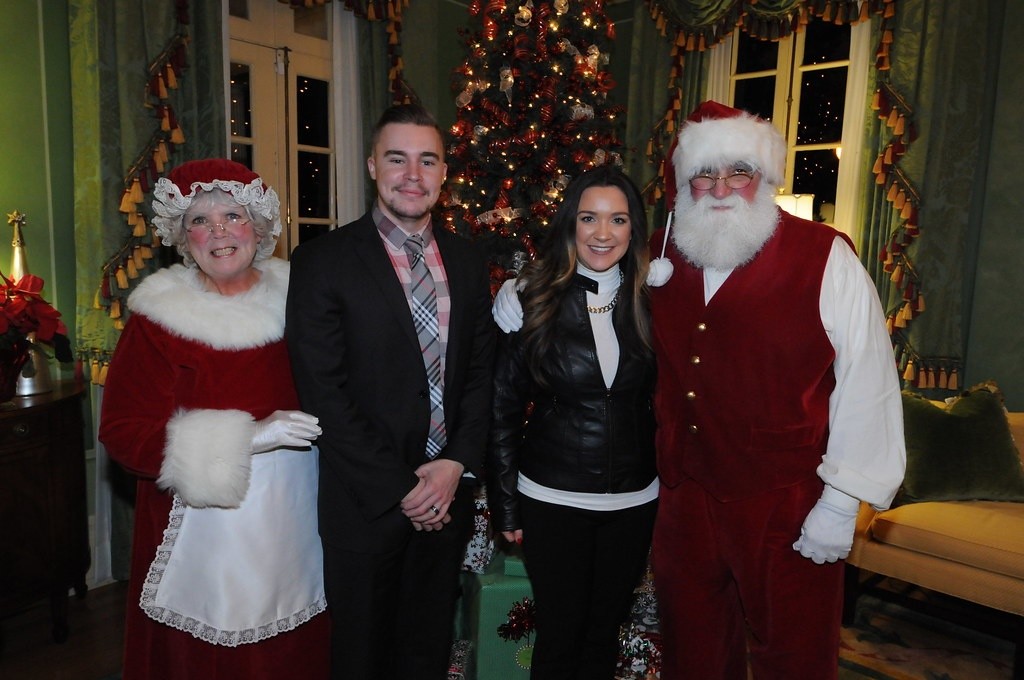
[152,158,282,246]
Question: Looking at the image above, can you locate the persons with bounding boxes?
[488,166,661,680]
[489,100,906,680]
[98,157,328,679]
[284,106,491,680]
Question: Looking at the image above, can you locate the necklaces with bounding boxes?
[585,271,627,314]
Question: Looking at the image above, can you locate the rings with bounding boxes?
[430,505,439,516]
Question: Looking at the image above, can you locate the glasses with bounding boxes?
[689,173,754,190]
[183,218,254,242]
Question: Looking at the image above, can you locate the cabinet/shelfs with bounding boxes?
[1,378,91,644]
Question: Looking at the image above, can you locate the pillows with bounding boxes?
[899,379,1024,503]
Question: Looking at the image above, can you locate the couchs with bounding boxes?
[840,412,1024,679]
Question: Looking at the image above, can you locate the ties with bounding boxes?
[403,235,448,459]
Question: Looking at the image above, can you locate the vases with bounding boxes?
[0,342,30,402]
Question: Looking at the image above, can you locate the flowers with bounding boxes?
[1,272,69,342]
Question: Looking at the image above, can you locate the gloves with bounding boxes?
[491,278,527,334]
[790,485,858,564]
[253,410,321,454]
